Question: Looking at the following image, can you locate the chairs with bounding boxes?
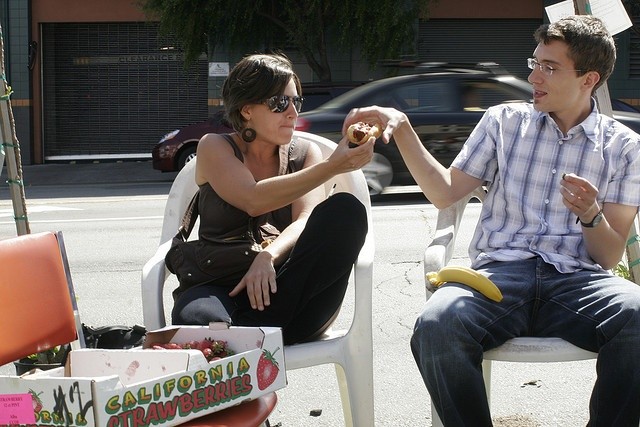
[1,230,277,425]
[141,127,377,426]
[423,181,599,427]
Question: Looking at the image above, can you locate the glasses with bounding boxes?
[251,95,303,113]
[527,58,581,76]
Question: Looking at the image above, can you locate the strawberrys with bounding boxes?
[256,347,281,390]
[149,336,236,363]
[27,388,44,412]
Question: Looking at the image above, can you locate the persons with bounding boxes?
[170,54,377,344]
[342,16,639,427]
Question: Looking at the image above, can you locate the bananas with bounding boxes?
[426,266,503,304]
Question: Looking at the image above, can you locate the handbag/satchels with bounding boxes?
[165,222,280,298]
[62,323,147,366]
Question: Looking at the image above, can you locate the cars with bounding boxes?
[294,60,640,201]
[153,106,237,171]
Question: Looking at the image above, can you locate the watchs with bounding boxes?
[575,209,603,227]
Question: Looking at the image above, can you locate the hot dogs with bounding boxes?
[346,118,384,146]
